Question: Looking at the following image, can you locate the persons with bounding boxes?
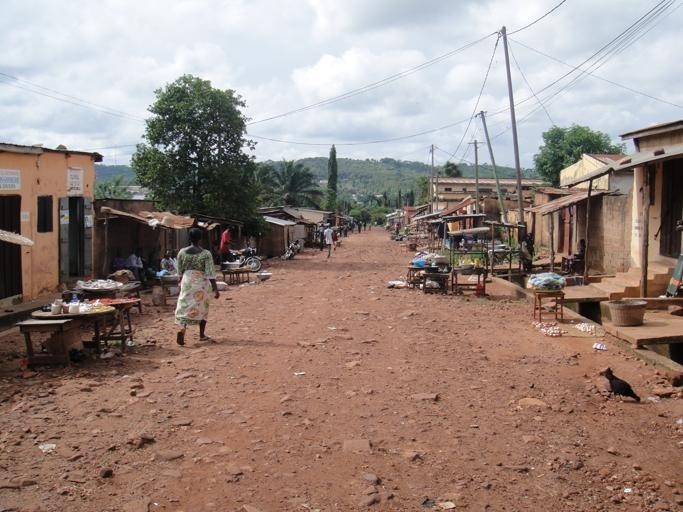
[574,238,586,258]
[220,225,235,271]
[114,247,180,281]
[175,227,220,345]
[319,220,372,259]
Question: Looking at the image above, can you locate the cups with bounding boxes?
[81,279,122,288]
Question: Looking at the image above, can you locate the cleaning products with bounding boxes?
[474,258,480,270]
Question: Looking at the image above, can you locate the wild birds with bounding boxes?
[599,366,641,402]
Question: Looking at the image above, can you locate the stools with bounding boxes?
[223,266,253,286]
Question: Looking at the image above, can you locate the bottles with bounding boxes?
[49,293,102,315]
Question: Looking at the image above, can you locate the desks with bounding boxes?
[531,290,566,323]
[15,279,142,370]
[159,273,179,306]
[404,248,488,301]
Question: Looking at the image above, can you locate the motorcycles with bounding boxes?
[227,242,262,272]
[280,241,300,260]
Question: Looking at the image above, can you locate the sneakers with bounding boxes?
[199,336,211,341]
[176,331,184,345]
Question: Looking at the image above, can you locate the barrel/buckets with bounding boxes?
[448,221,460,232]
[151,285,164,306]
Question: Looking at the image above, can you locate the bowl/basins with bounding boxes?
[256,273,272,280]
[424,266,440,273]
[214,281,227,291]
[460,263,474,270]
